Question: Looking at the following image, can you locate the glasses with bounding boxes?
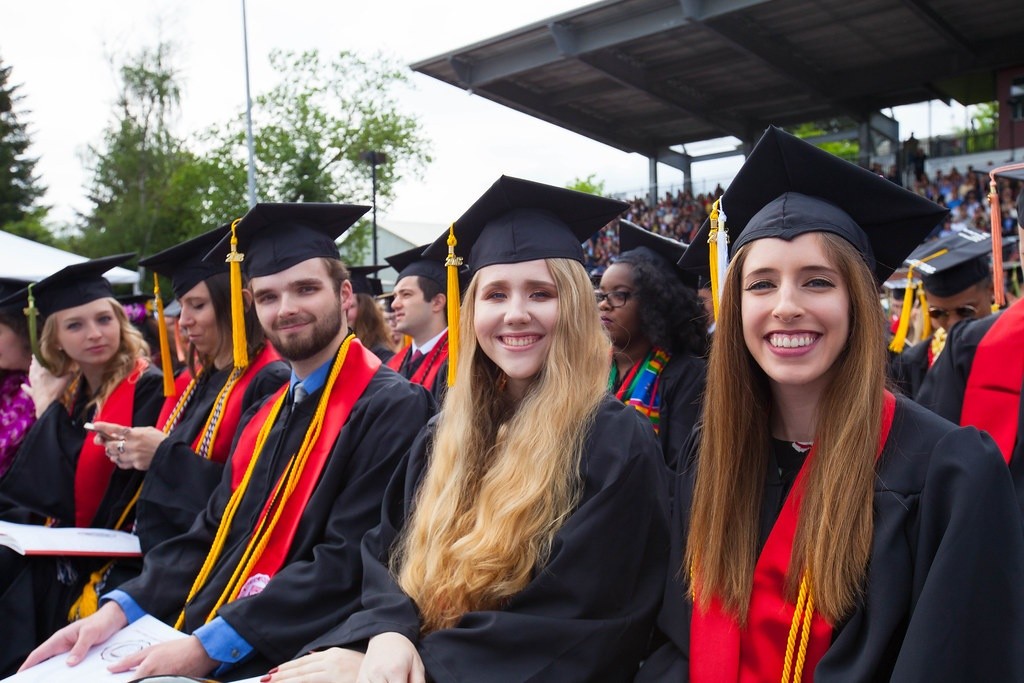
[929,305,976,319]
[596,290,632,307]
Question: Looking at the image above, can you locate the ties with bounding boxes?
[289,380,307,412]
[407,349,424,373]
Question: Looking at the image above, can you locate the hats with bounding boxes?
[202,202,372,373]
[385,242,448,290]
[674,124,950,331]
[886,226,1024,353]
[0,252,138,373]
[422,174,630,392]
[348,265,390,296]
[613,220,686,271]
[139,223,245,399]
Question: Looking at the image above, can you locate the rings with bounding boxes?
[117,443,124,453]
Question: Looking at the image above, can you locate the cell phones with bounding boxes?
[84,422,120,441]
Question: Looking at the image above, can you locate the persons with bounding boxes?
[0,125,1024,683]
